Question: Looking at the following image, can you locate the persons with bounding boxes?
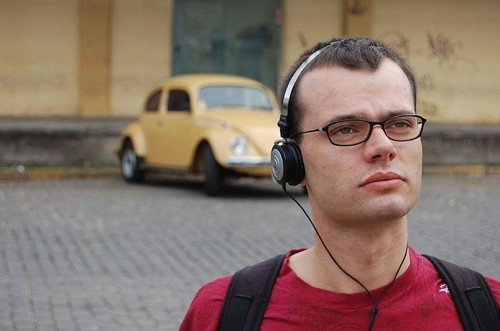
[176,35,500,331]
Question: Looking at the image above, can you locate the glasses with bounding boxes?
[289,114,427,147]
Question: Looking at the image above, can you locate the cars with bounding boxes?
[114,72,282,195]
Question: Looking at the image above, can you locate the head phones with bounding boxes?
[270,41,339,186]
